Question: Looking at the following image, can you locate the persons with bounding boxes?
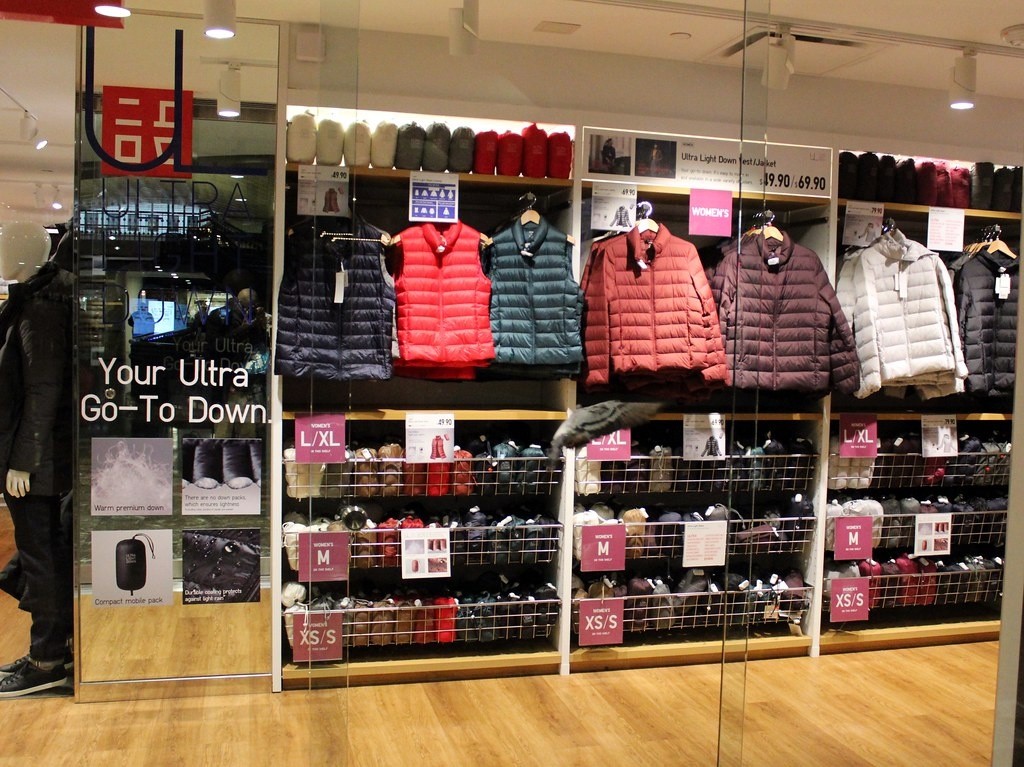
[0,214,72,699]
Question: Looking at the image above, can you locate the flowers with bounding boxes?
[382,194,1018,260]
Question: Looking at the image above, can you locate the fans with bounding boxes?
[719,30,869,57]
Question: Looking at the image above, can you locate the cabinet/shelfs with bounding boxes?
[271,99,1024,694]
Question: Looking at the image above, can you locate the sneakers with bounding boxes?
[0,653,74,676]
[0,660,67,697]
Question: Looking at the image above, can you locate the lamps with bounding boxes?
[761,22,801,93]
[202,1,236,40]
[450,0,480,57]
[950,48,983,110]
[216,62,242,117]
